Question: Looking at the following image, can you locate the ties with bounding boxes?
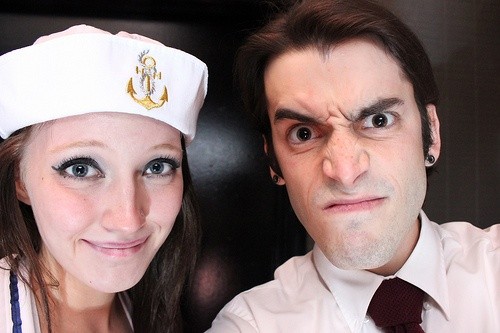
[364,278,431,333]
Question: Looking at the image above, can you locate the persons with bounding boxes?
[0,24,208,333]
[206,0,500,333]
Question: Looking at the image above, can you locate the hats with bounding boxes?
[0,24,208,143]
